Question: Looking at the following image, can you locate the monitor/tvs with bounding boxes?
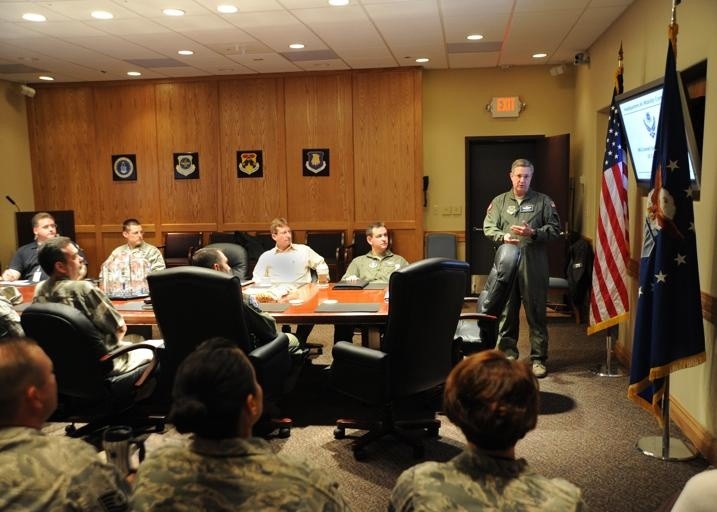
[615,72,701,197]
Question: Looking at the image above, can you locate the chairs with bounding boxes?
[545,240,593,327]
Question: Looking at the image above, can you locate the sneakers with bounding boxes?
[531,360,547,377]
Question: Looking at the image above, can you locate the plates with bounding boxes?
[257,285,273,288]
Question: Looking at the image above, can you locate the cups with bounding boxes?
[101,252,147,298]
[259,276,270,284]
[318,271,329,289]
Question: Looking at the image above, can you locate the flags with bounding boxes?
[586,64,630,338]
[628,38,707,428]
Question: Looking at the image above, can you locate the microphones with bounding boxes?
[6,196,21,212]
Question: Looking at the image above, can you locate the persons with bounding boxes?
[192,247,305,394]
[0,295,25,340]
[100,219,166,282]
[483,160,562,378]
[0,335,133,512]
[31,237,165,376]
[386,350,587,512]
[252,218,331,344]
[133,338,354,512]
[1,212,89,283]
[334,221,410,344]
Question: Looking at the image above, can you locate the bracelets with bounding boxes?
[531,230,537,240]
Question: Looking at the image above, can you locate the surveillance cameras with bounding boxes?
[574,53,584,61]
